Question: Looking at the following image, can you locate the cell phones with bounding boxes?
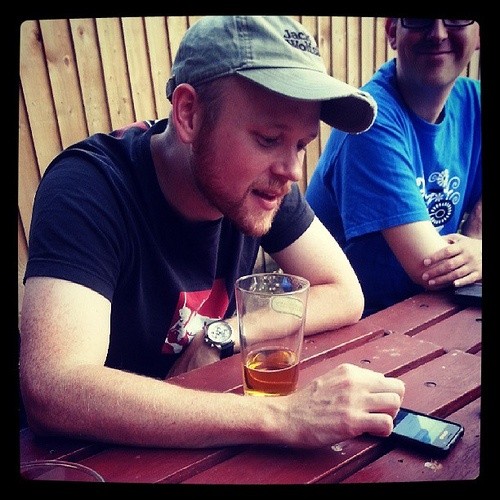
[452,282,482,306]
[371,407,465,458]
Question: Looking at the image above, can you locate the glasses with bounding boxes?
[400,17,475,30]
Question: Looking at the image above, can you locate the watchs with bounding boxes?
[202,318,234,361]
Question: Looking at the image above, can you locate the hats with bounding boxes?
[166,16,378,134]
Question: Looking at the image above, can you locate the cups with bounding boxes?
[20,460,105,482]
[234,273,310,397]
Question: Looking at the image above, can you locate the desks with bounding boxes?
[18,289,481,486]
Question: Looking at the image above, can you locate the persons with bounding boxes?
[304,17,482,321]
[18,16,406,450]
